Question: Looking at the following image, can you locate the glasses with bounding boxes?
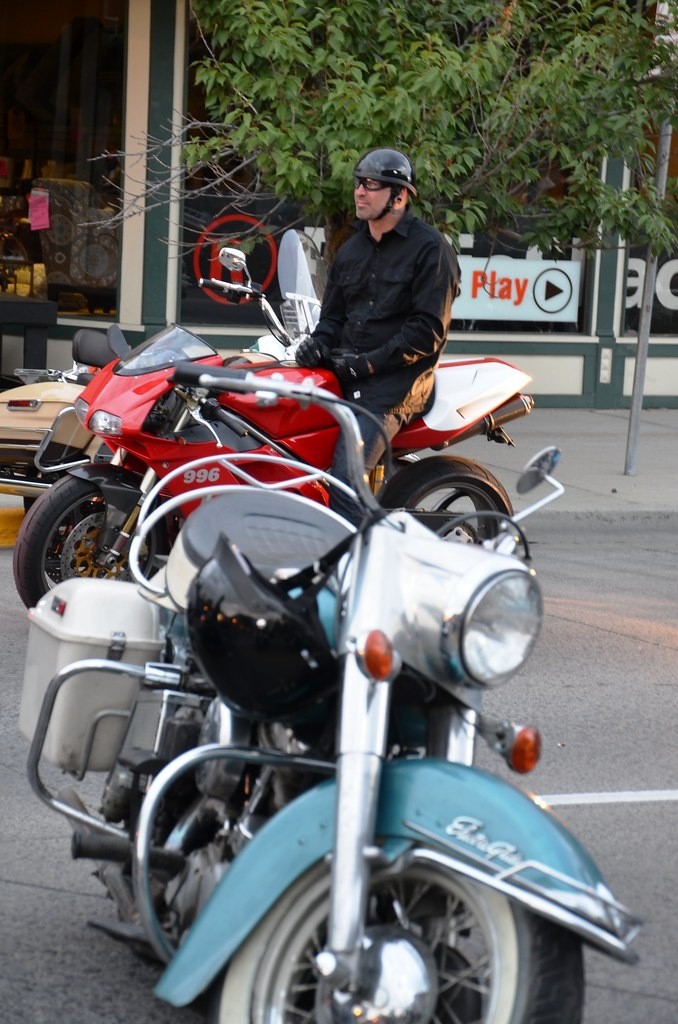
[353,176,394,192]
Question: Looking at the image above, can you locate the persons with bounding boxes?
[294,146,462,533]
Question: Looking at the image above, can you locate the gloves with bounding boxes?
[327,348,370,384]
[295,336,329,368]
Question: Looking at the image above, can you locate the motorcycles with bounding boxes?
[0,229,642,1023]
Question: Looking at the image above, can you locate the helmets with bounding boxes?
[352,148,417,198]
[187,532,344,725]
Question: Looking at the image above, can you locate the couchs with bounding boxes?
[33,178,118,315]
